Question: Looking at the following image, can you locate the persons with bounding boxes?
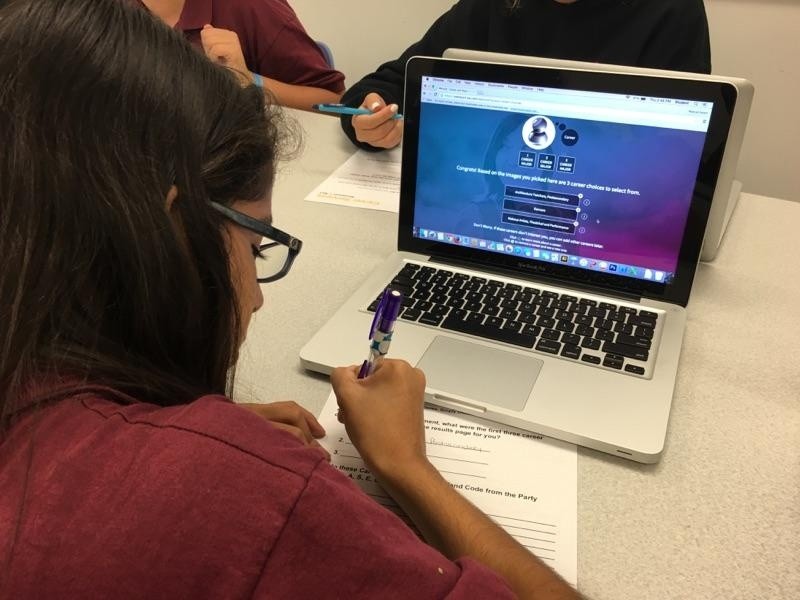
[0,0,592,599]
[338,0,713,152]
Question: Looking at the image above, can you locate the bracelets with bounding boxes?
[253,73,262,90]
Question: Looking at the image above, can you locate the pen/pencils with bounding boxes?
[357,288,402,379]
[312,103,402,119]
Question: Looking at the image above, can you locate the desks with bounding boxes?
[226,101,800,600]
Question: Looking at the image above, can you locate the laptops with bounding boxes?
[298,47,752,466]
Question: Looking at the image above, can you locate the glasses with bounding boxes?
[202,197,303,283]
